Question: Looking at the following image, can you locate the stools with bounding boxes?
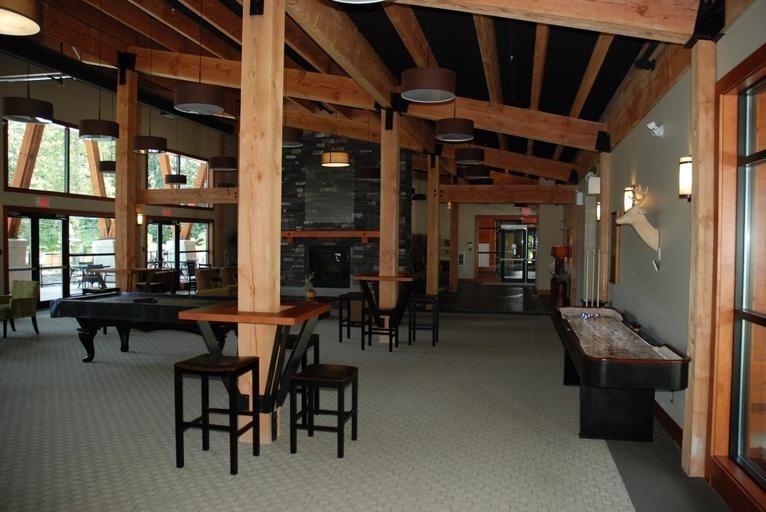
[175,334,357,475]
[339,292,440,352]
[82,288,120,335]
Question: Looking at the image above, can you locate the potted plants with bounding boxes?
[302,271,317,302]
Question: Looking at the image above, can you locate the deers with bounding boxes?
[615,184,659,251]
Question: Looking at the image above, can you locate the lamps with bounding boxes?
[550,244,571,312]
[284,105,381,182]
[678,156,693,203]
[401,42,492,200]
[622,183,634,212]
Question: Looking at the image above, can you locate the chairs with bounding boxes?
[69,251,238,302]
[0,280,40,338]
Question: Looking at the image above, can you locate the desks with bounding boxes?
[352,273,422,327]
[178,301,332,412]
[548,307,690,441]
[50,291,237,362]
